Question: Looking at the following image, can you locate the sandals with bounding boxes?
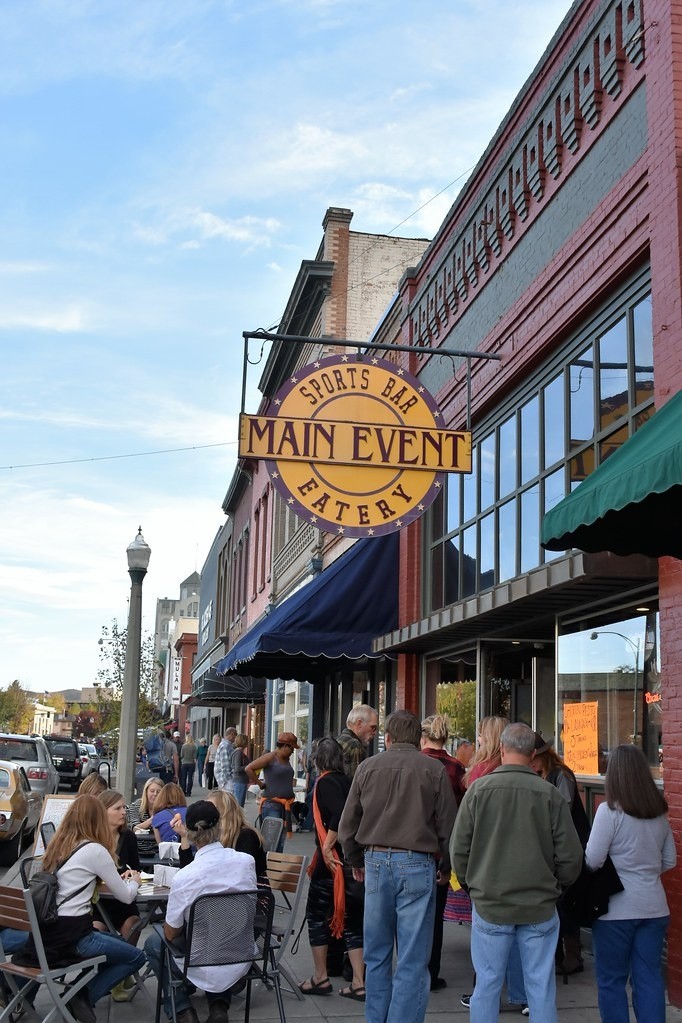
[298,975,332,995]
[339,984,366,1001]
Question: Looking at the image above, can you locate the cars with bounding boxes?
[51,743,93,781]
[0,759,42,868]
[78,743,101,775]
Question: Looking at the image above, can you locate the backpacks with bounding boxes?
[143,734,172,772]
[28,841,97,926]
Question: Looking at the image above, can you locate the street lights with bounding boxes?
[114,521,155,809]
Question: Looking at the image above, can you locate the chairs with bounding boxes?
[250,849,310,1001]
[0,885,107,1023]
[20,821,112,998]
[152,889,285,1023]
[260,815,292,911]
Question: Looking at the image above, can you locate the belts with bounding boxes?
[366,845,408,852]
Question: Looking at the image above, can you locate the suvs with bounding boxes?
[0,731,60,800]
[41,732,87,792]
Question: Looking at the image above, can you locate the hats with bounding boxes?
[173,731,180,737]
[534,732,550,755]
[277,732,299,749]
[185,800,219,831]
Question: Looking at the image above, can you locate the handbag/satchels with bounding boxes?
[202,770,207,787]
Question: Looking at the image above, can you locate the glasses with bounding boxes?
[372,728,376,732]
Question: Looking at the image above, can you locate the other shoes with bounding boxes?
[555,963,584,975]
[59,981,96,1023]
[111,975,136,1002]
[431,979,446,991]
[0,982,36,1013]
[460,994,472,1007]
[521,1003,530,1015]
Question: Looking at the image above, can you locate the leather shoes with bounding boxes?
[169,1009,200,1023]
[204,1003,229,1023]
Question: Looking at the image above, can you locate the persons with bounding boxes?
[450,723,583,1023]
[27,794,146,1023]
[584,743,677,1023]
[292,736,312,824]
[255,749,270,805]
[136,742,146,765]
[460,715,511,1007]
[110,742,118,771]
[299,738,320,833]
[326,704,379,982]
[295,735,366,1001]
[181,791,275,995]
[229,734,250,808]
[145,800,258,1023]
[0,834,43,1023]
[130,777,164,874]
[133,782,196,873]
[337,709,458,1023]
[418,714,467,991]
[530,732,592,976]
[245,732,300,874]
[76,772,107,796]
[146,727,238,797]
[93,789,141,990]
[97,740,106,755]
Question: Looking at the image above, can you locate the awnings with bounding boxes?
[200,530,399,704]
[541,389,682,561]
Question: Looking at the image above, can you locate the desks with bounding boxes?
[248,785,307,828]
[139,852,179,921]
[137,834,155,842]
[95,878,174,1023]
